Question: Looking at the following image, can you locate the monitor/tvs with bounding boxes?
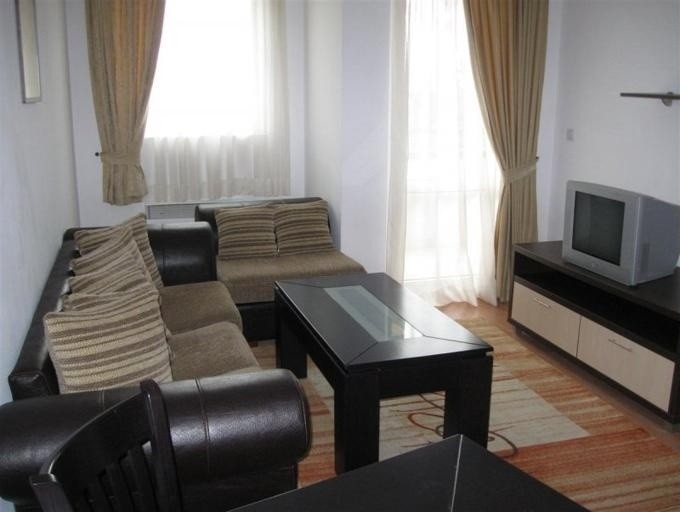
[561,179,680,286]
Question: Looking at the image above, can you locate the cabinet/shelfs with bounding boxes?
[506,241,678,425]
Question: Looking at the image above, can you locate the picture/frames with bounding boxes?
[15,0,42,103]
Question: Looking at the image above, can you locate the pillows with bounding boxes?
[213,198,338,261]
[43,214,173,393]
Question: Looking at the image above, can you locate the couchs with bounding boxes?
[1,212,313,512]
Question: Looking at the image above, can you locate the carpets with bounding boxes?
[250,318,680,509]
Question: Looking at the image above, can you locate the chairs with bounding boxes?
[194,196,367,349]
